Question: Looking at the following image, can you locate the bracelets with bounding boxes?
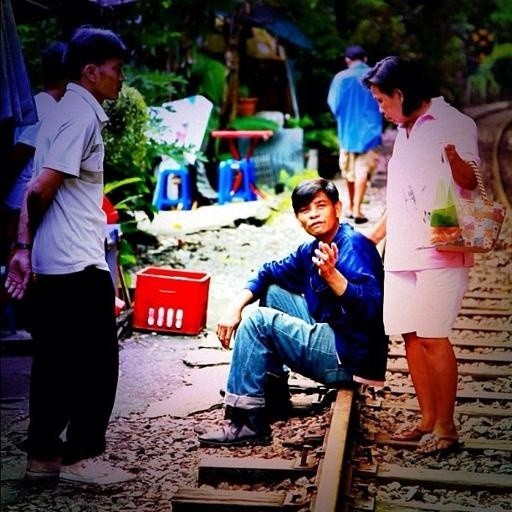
[12,240,31,250]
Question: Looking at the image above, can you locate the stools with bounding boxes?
[151,161,196,210]
[216,158,258,205]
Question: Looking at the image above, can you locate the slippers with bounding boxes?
[391,421,431,441]
[416,434,464,454]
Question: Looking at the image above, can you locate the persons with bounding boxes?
[361,55,481,457]
[199,178,388,445]
[5,25,138,490]
[326,44,383,223]
[1,40,71,338]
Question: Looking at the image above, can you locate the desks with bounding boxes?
[211,130,274,202]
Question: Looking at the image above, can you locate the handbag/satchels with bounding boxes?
[435,160,507,253]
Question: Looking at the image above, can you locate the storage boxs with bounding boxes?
[133,266,211,336]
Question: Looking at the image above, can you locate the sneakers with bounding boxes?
[26,458,62,477]
[59,456,136,484]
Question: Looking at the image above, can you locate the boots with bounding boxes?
[220,370,291,412]
[198,408,271,446]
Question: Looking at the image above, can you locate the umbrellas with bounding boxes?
[0,1,39,125]
